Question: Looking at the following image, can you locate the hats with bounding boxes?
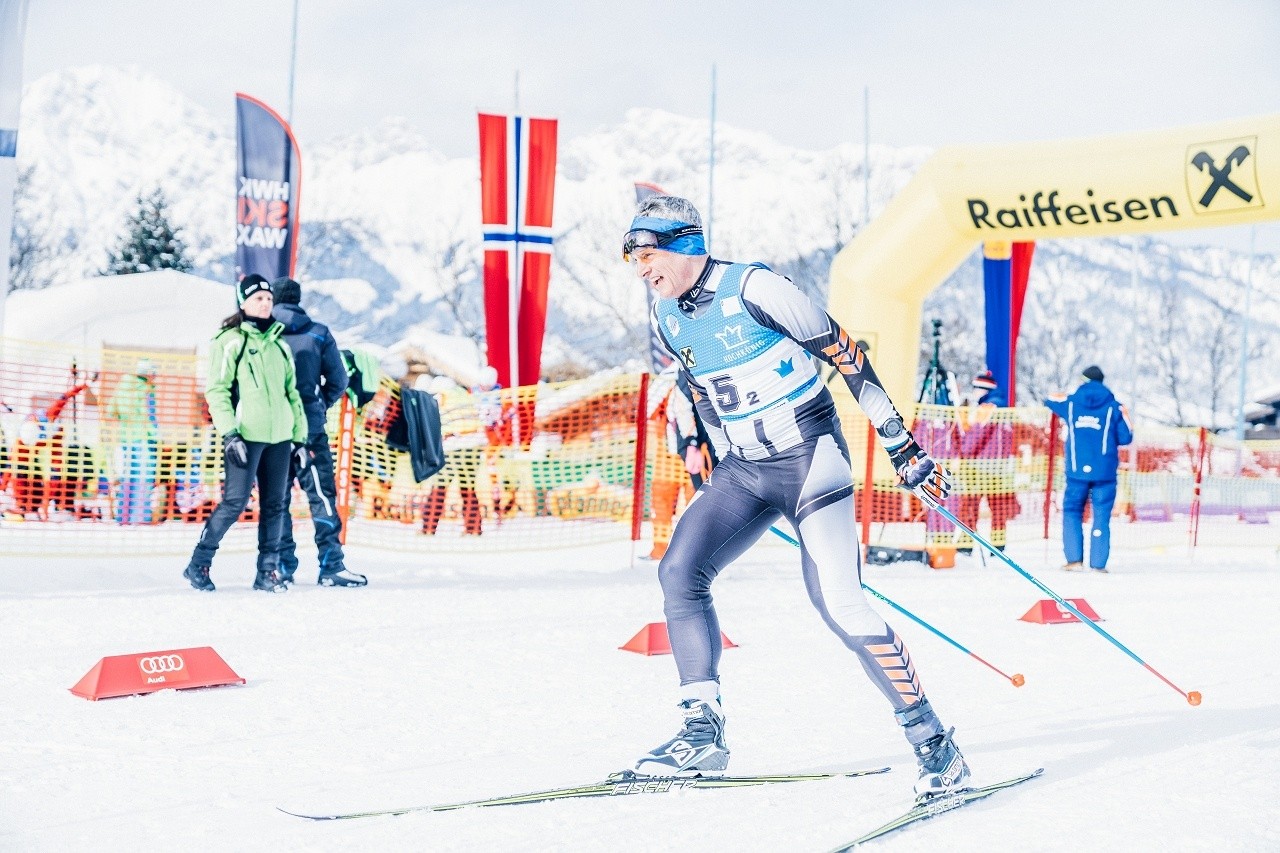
[1081,366,1104,383]
[972,371,998,390]
[236,273,273,307]
[271,276,301,305]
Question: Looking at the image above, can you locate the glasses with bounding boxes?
[622,228,659,262]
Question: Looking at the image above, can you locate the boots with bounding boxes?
[635,694,731,774]
[894,694,971,794]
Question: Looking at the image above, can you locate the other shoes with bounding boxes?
[1092,568,1108,573]
[957,548,972,556]
[1060,561,1083,571]
[990,546,1005,557]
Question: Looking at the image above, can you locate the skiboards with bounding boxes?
[274,761,1047,853]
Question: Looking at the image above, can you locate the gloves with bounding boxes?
[224,433,248,468]
[291,442,307,469]
[888,430,952,509]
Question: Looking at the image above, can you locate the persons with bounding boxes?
[1038,366,1135,573]
[621,195,971,802]
[105,275,489,594]
[952,371,1012,555]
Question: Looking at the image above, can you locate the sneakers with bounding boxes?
[318,569,367,588]
[278,573,294,586]
[253,570,288,592]
[183,561,216,592]
